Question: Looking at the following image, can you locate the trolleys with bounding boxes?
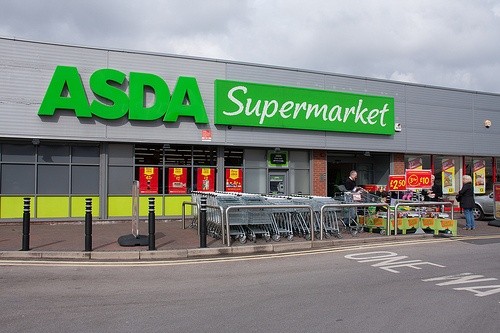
[191,188,363,243]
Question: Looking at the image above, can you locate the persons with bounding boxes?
[343,169,361,230]
[456,174,476,230]
[390,175,443,203]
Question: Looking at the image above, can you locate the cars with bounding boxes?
[473,192,494,219]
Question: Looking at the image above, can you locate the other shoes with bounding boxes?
[455,192,463,202]
[462,226,476,231]
